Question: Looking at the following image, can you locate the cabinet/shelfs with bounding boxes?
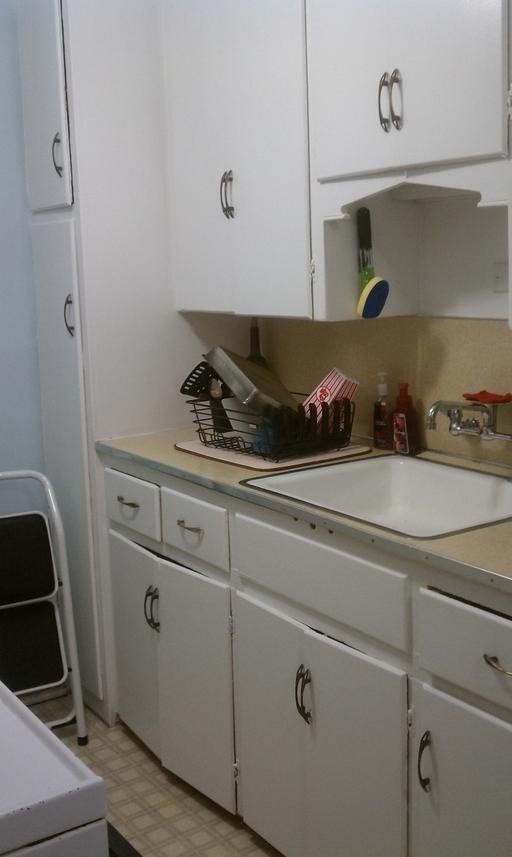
[16,1,278,730]
[155,0,416,328]
[309,1,509,185]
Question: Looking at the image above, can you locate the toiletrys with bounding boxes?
[371,369,391,449]
[388,380,421,454]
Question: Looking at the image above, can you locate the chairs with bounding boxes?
[0,467,87,750]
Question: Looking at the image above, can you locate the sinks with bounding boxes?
[239,444,512,542]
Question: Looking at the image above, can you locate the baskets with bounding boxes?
[185,392,355,463]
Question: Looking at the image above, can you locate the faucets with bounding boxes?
[422,400,496,439]
